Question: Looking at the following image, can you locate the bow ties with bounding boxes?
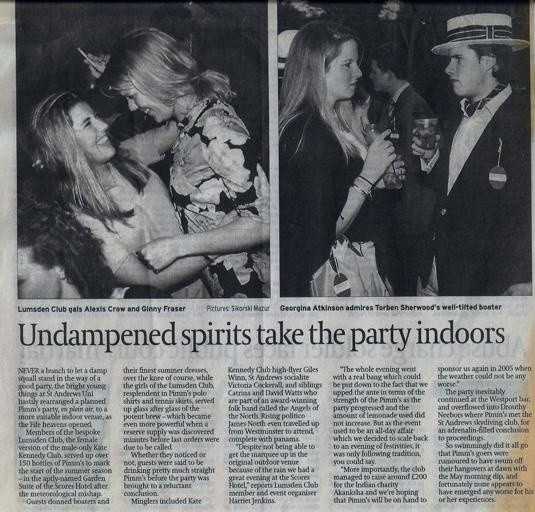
[459,79,509,120]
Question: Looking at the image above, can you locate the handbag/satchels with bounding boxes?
[309,238,391,296]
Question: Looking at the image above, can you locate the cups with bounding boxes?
[415,118,439,150]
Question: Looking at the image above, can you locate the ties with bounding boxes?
[386,100,397,133]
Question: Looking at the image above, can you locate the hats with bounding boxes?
[429,11,531,57]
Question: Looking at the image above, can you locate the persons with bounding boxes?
[17,202,128,298]
[368,50,439,236]
[417,11,531,296]
[87,30,270,298]
[280,19,405,296]
[33,92,265,298]
